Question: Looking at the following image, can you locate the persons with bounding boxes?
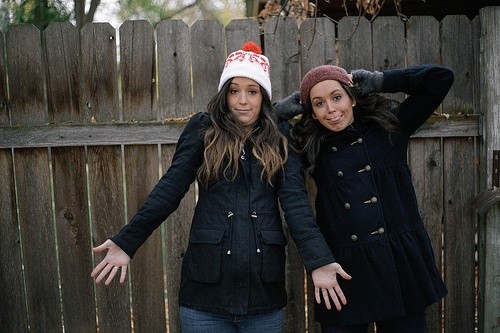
[272,65,455,333]
[91,42,352,333]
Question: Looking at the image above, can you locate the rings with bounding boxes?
[114,266,119,270]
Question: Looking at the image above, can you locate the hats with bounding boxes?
[299,65,352,111]
[218,42,272,100]
